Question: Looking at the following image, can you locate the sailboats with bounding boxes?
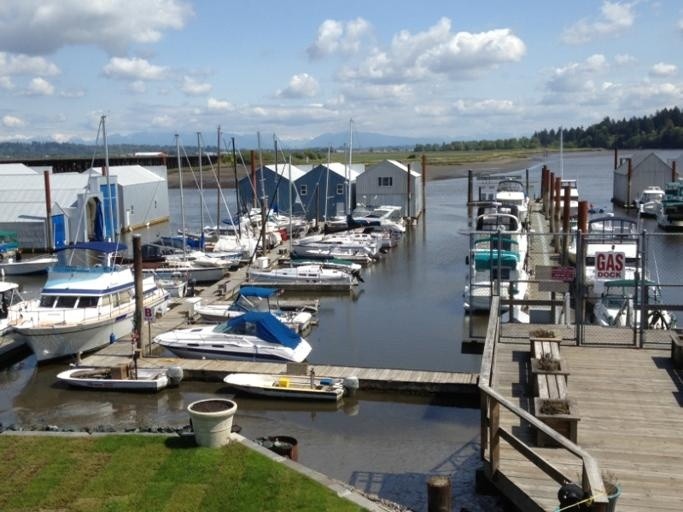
[6,114,172,356]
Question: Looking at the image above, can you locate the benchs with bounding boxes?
[528,328,580,448]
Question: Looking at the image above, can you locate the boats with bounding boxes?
[0,267,26,355]
[51,385,184,416]
[11,361,82,416]
[153,310,313,364]
[0,230,60,276]
[53,363,167,393]
[463,171,683,329]
[222,371,343,404]
[192,287,313,331]
[110,117,408,299]
[220,387,360,425]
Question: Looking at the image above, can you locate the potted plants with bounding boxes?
[604,478,621,512]
